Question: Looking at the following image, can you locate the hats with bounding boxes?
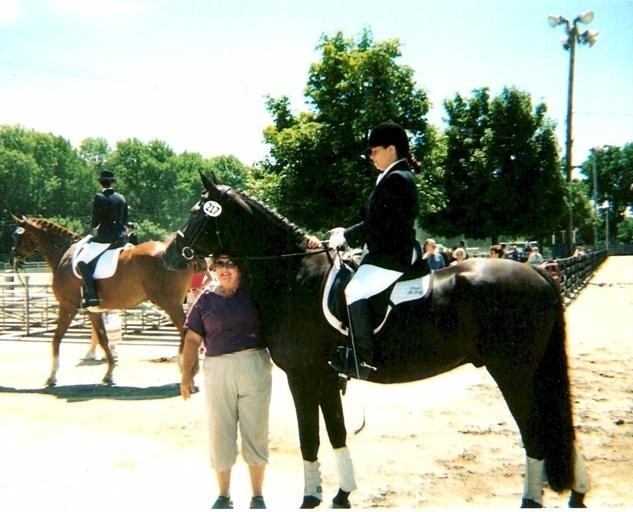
[452,247,466,259]
[361,120,408,154]
[97,171,115,183]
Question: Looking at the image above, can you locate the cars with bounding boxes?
[501,240,538,254]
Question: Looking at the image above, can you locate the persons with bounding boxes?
[78,313,118,362]
[422,238,445,270]
[449,241,544,267]
[125,222,138,246]
[186,270,212,311]
[73,171,129,309]
[573,246,586,256]
[179,234,322,509]
[327,121,422,380]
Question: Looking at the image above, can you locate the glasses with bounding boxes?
[216,259,235,269]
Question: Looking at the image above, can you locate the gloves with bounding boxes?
[329,230,346,250]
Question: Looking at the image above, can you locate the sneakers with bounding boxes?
[251,495,266,508]
[211,496,233,509]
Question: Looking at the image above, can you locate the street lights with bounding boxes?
[545,10,600,183]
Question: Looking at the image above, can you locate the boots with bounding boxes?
[328,298,378,379]
[75,261,101,311]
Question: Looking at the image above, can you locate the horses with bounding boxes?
[9,212,200,390]
[162,168,589,509]
